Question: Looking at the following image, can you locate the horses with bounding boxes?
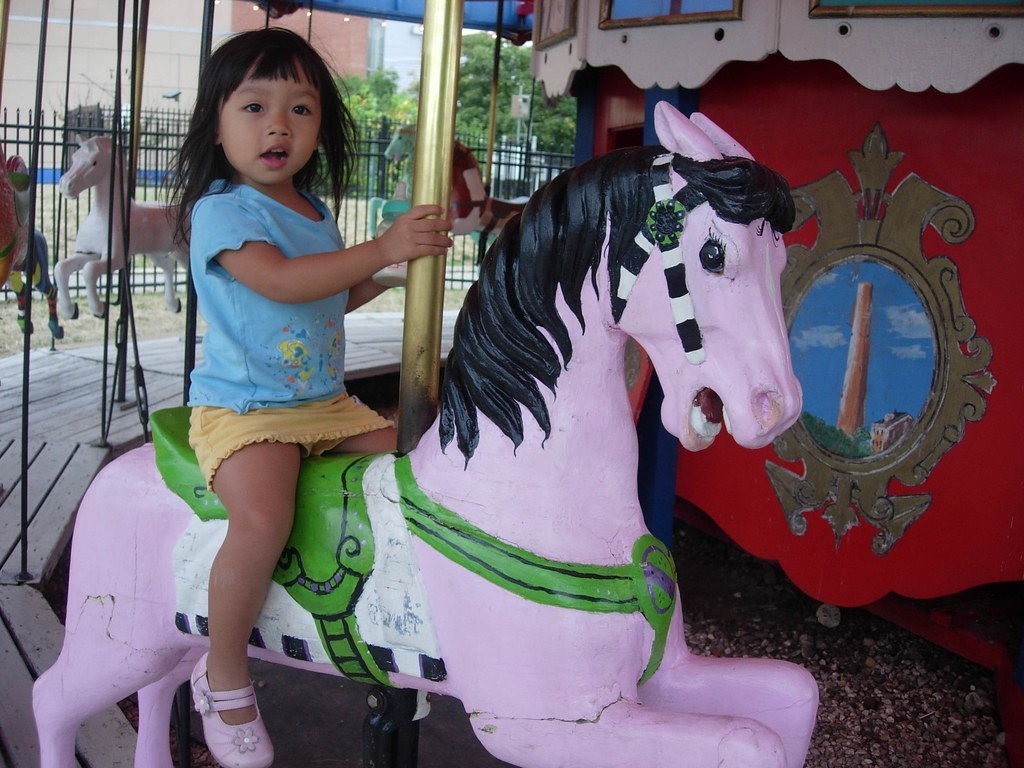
[367,123,529,265]
[0,147,64,340]
[29,103,821,767]
[53,133,192,320]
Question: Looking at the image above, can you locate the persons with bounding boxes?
[163,27,456,768]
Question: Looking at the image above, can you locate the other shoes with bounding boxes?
[190,651,275,767]
